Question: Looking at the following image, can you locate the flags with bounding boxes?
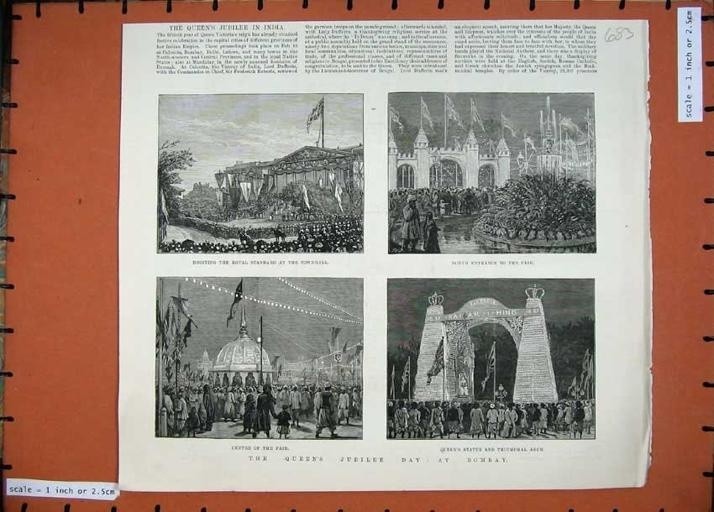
[307,98,324,133]
[427,340,444,385]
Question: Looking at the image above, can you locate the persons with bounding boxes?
[387,399,592,439]
[162,381,363,438]
[388,185,498,253]
[497,384,509,401]
[162,214,364,252]
